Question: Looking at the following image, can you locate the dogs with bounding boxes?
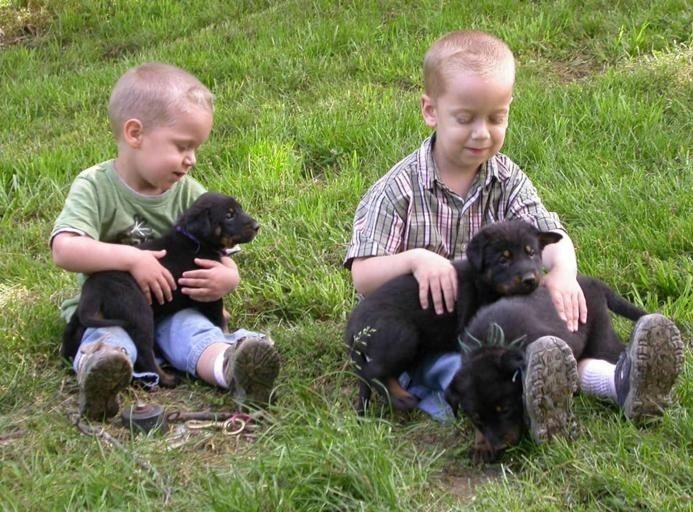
[340,216,562,416]
[442,273,647,470]
[56,190,262,389]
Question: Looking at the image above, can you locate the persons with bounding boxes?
[342,30,684,451]
[47,61,281,423]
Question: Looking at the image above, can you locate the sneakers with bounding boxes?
[614,312,684,431]
[223,337,279,408]
[522,334,578,448]
[80,337,131,421]
